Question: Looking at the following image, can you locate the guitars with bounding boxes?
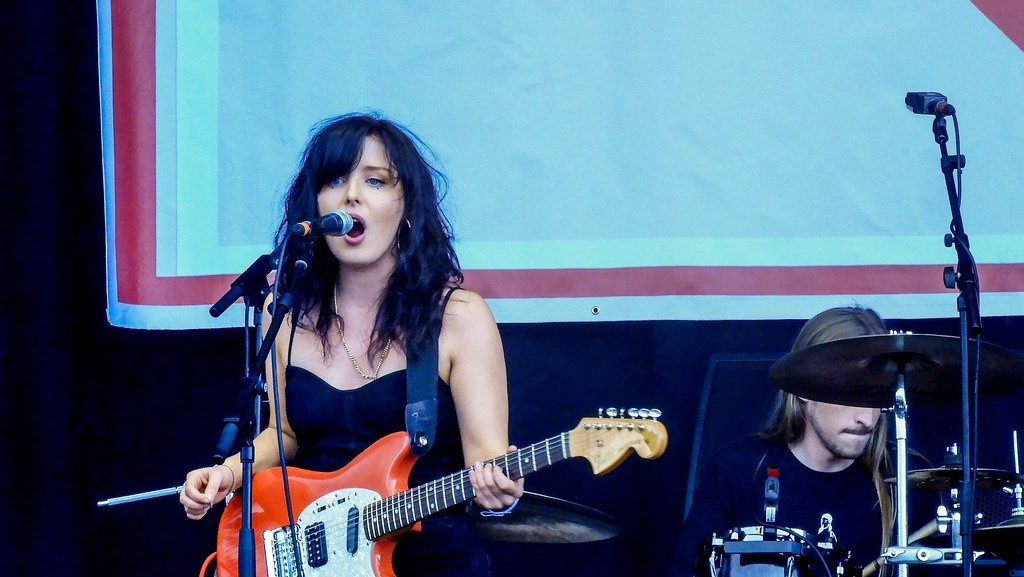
[215,407,670,577]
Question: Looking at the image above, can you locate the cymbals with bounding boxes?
[470,489,624,542]
[767,333,1024,409]
[881,465,1022,491]
[931,516,1024,563]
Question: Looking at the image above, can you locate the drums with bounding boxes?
[697,525,851,577]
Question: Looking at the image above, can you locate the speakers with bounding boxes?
[680,350,787,525]
[970,457,1019,532]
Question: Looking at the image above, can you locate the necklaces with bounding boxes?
[334,280,391,379]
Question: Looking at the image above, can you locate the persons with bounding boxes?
[180,110,525,577]
[669,307,1009,577]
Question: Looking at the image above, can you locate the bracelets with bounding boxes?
[213,464,234,496]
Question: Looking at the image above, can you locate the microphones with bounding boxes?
[761,450,781,524]
[292,209,354,236]
[905,91,956,115]
